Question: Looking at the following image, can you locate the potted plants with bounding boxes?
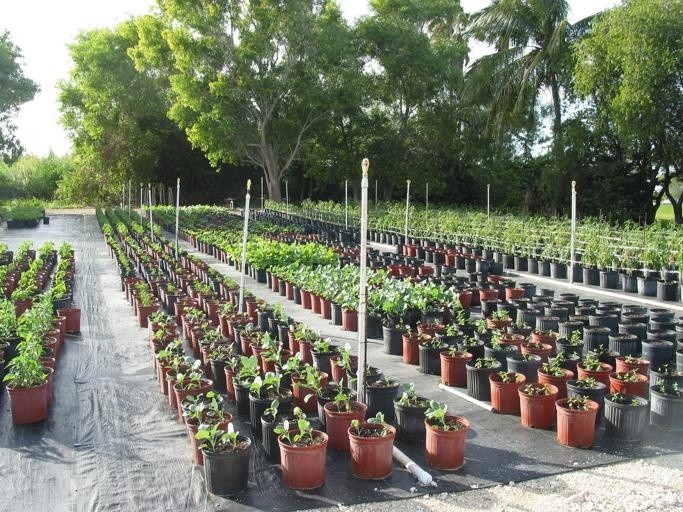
[1,248,81,425]
[7,210,51,230]
